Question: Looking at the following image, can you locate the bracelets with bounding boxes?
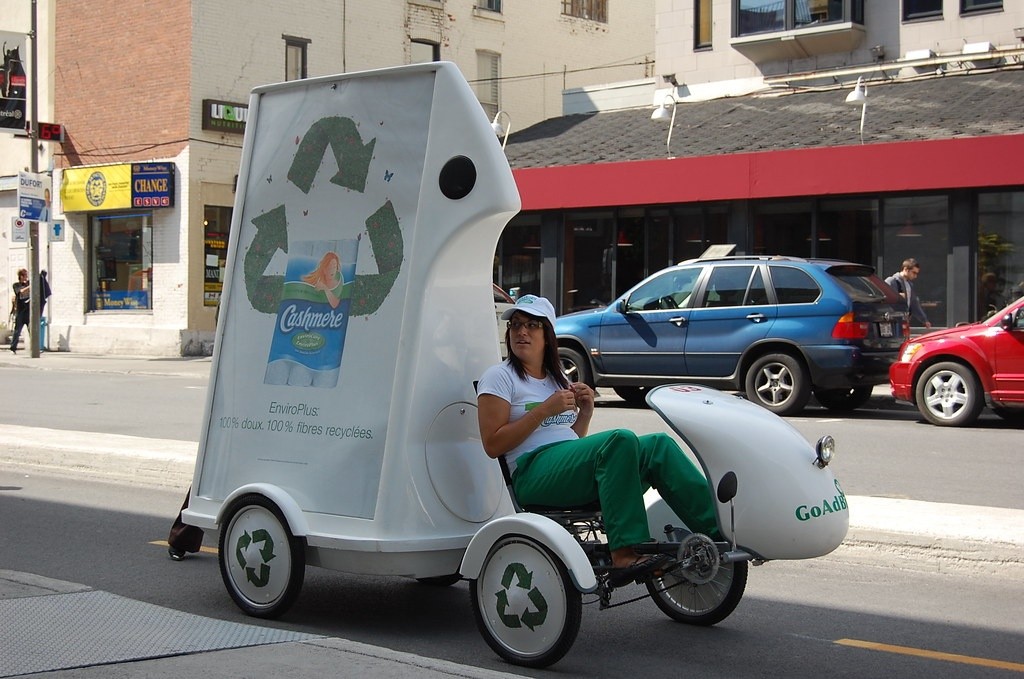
[527,411,540,425]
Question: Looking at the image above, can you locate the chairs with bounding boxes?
[474,379,606,522]
[709,278,739,307]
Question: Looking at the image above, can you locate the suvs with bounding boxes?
[555,252,910,418]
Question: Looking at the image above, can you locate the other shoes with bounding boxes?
[627,554,664,568]
[40,350,44,353]
[11,350,16,355]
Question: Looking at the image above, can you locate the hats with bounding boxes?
[501,295,556,330]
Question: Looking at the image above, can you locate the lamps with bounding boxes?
[689,227,710,243]
[905,48,937,67]
[869,44,886,63]
[845,75,870,143]
[523,234,541,249]
[651,94,678,161]
[661,74,679,87]
[1014,27,1024,41]
[491,110,512,154]
[895,213,923,236]
[962,42,997,61]
[610,230,633,246]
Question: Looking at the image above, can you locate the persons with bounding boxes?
[477,295,729,570]
[982,273,1006,313]
[885,258,931,329]
[10,269,30,355]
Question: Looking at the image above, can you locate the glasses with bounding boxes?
[507,320,543,329]
[911,271,919,275]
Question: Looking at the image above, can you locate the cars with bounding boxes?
[491,283,518,360]
[890,294,1024,428]
